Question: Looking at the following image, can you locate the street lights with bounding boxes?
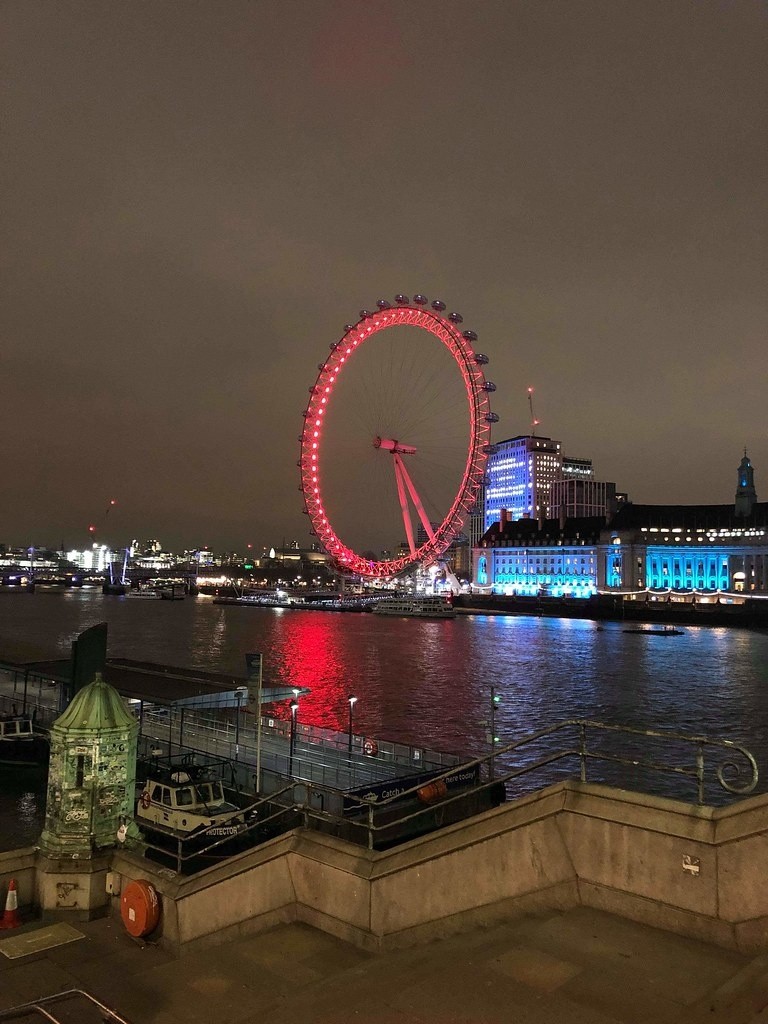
[288,701,299,775]
[347,694,357,767]
[291,686,301,754]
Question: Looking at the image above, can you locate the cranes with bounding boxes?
[88,501,115,542]
[527,386,540,437]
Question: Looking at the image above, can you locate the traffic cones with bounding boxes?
[0,877,22,929]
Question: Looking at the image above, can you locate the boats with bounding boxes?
[213,592,456,618]
[0,715,49,767]
[132,753,256,854]
[623,626,685,636]
[128,583,186,600]
